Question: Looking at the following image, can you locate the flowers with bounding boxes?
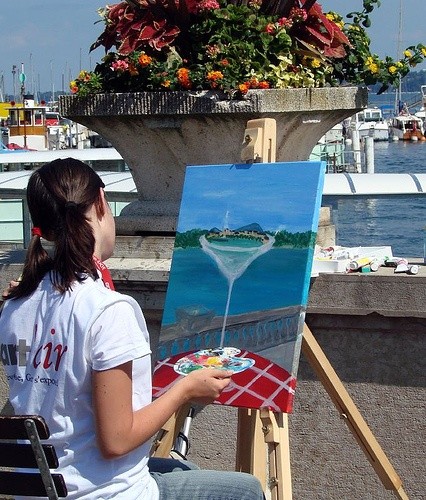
[66,0,426,97]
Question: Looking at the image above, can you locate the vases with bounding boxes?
[58,88,367,231]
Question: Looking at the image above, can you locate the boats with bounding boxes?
[309,0,426,171]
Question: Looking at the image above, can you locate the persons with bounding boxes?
[0,158,266,500]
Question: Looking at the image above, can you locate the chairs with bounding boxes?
[0,414,69,500]
